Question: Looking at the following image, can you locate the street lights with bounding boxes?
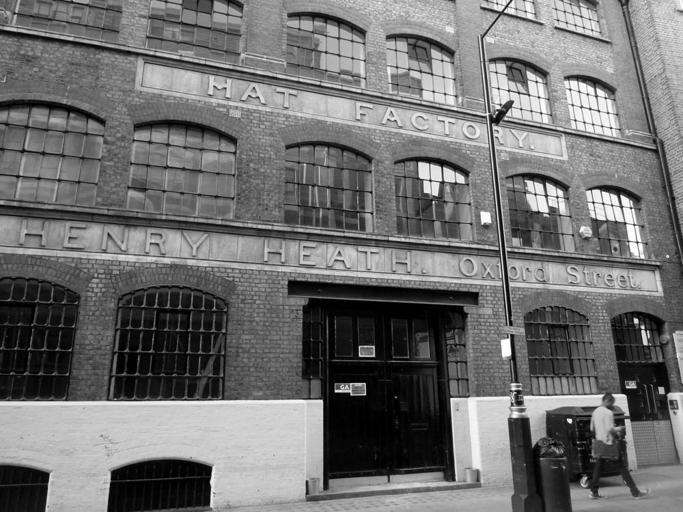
[476,0,546,512]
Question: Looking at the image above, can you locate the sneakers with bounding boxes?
[589,491,605,499]
[633,489,648,499]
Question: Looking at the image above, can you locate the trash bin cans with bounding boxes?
[540,458,573,512]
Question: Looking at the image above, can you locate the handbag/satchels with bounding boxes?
[591,438,620,461]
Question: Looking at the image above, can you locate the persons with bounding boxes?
[588,392,650,501]
